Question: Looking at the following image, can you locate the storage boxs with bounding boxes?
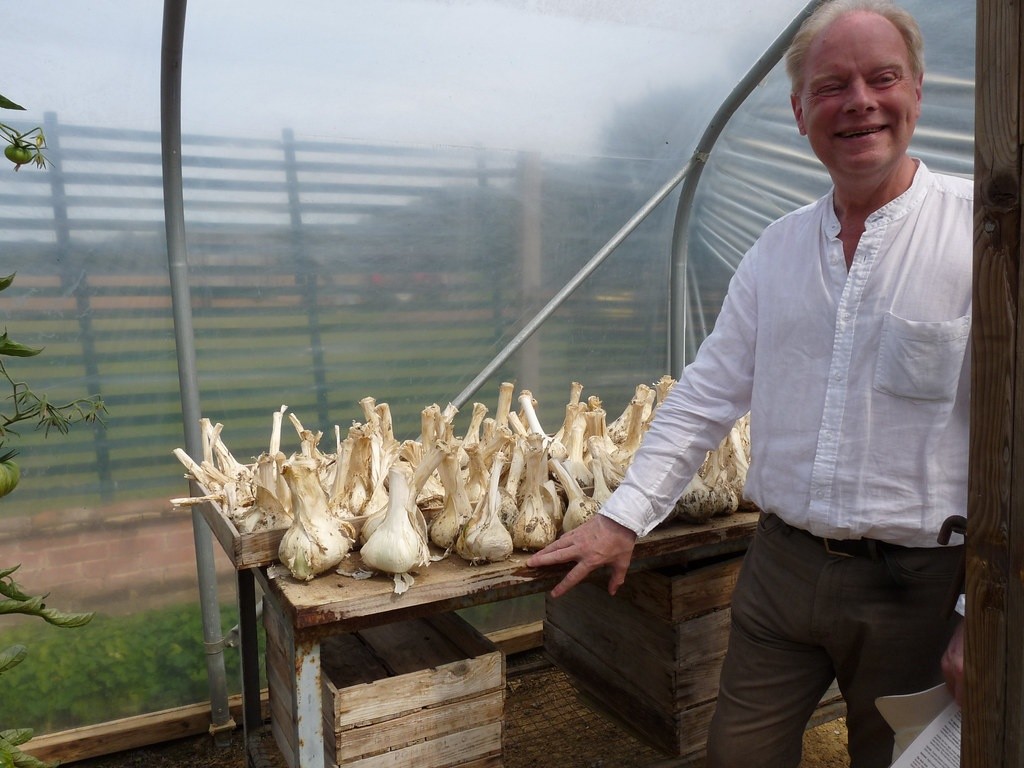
[262,589,507,768]
[188,478,373,572]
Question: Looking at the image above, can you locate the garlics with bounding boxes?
[170,376,759,581]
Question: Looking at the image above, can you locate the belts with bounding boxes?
[801,529,965,559]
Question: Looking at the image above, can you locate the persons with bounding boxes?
[525,0,975,768]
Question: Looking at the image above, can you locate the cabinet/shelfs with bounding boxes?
[236,508,849,768]
